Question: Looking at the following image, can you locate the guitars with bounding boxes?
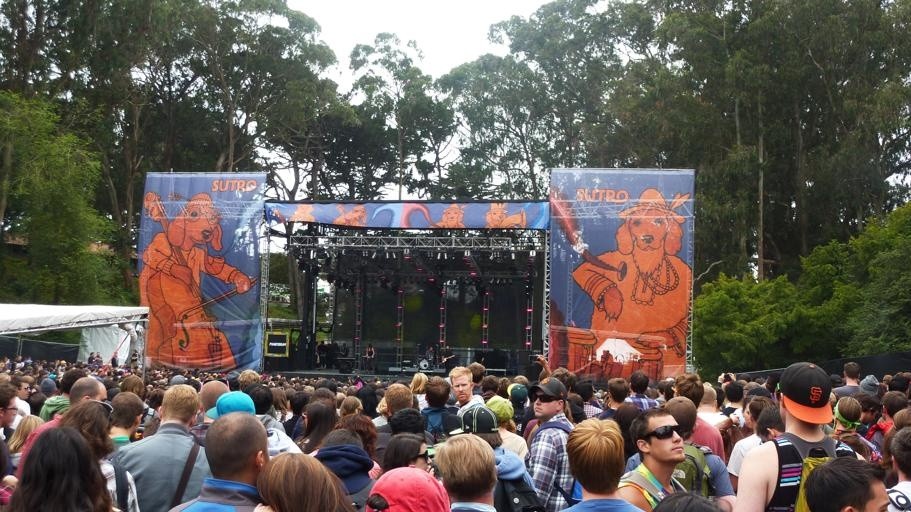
[442,354,455,362]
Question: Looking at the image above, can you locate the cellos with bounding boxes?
[144,191,236,370]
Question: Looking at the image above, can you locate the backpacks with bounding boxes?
[493,477,548,511]
[779,431,838,512]
[669,442,717,500]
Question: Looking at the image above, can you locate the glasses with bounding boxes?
[88,400,114,429]
[418,450,429,463]
[642,423,691,440]
[530,392,561,403]
[201,379,230,391]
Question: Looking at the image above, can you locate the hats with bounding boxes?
[206,390,256,420]
[507,382,528,405]
[448,405,499,434]
[484,394,515,424]
[171,374,188,384]
[365,464,451,512]
[527,377,571,402]
[780,361,832,424]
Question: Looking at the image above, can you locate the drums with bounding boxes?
[600,340,664,383]
[419,358,429,369]
[566,328,598,380]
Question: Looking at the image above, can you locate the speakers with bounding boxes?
[518,350,542,381]
[264,356,290,371]
[475,350,507,376]
[433,369,446,377]
[292,350,312,370]
[389,367,402,374]
[339,365,352,374]
[402,368,418,375]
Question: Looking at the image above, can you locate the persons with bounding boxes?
[443,345,455,373]
[342,343,350,358]
[1,351,911,510]
[317,341,328,369]
[366,344,376,374]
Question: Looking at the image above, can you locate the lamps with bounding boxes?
[284,225,539,300]
